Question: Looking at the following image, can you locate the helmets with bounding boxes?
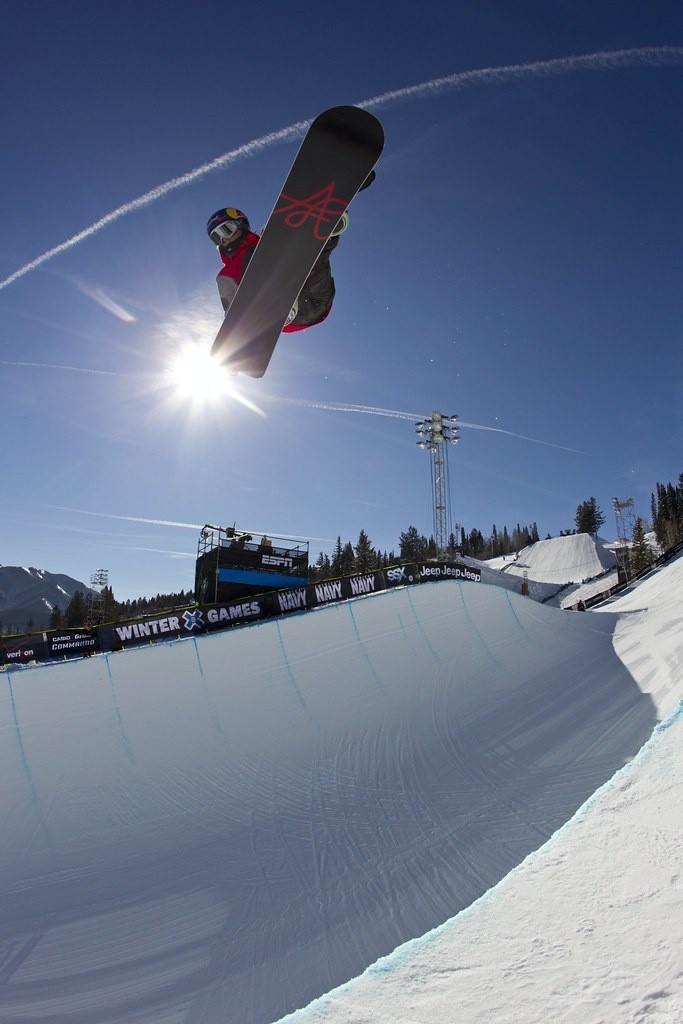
[206,208,249,245]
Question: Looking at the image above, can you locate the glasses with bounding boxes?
[210,220,238,246]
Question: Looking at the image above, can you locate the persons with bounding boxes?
[206,170,376,333]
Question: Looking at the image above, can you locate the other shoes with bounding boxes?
[284,301,299,327]
[331,212,349,237]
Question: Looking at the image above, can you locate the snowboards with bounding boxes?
[208,103,386,380]
